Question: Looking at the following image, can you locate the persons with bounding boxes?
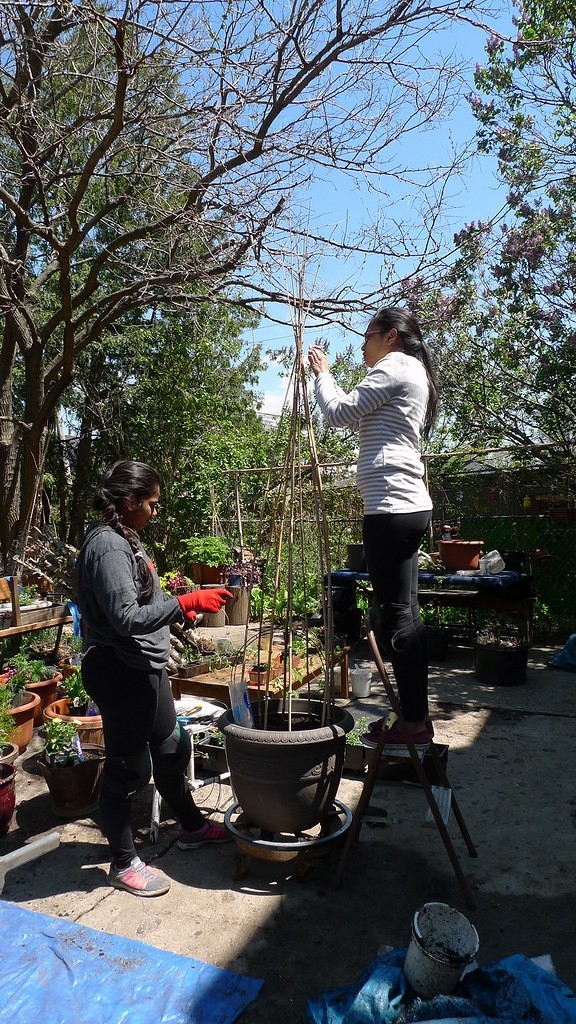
[74,461,234,897]
[307,308,436,750]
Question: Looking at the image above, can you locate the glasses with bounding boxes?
[365,327,400,343]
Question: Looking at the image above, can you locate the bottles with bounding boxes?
[226,680,255,729]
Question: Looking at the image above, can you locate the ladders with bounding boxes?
[330,606,477,910]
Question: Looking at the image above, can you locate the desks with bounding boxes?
[324,568,523,662]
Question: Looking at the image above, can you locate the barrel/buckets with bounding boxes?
[300,613,323,653]
[176,585,200,596]
[333,667,350,693]
[227,586,251,625]
[436,540,484,569]
[352,669,372,697]
[202,584,226,627]
[346,545,367,572]
[402,902,479,996]
[481,550,505,574]
[257,634,274,650]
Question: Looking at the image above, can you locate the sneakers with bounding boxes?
[368,709,434,739]
[360,721,431,751]
[106,856,170,896]
[176,818,239,850]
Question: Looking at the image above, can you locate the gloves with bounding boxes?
[177,589,234,613]
[183,611,196,623]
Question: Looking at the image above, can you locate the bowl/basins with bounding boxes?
[431,552,483,559]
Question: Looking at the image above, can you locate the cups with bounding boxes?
[479,560,491,575]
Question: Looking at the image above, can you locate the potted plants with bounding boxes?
[346,533,368,573]
[179,628,322,686]
[433,525,486,572]
[472,626,527,685]
[0,626,107,833]
[178,533,264,586]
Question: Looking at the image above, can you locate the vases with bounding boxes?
[217,697,355,833]
